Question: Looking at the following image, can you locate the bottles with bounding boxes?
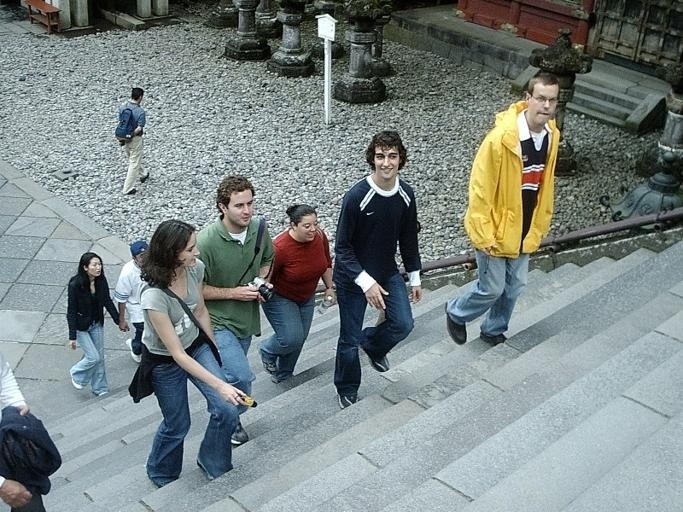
[317,296,332,314]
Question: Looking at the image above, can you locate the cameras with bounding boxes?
[240,276,275,303]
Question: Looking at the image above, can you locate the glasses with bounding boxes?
[531,95,558,105]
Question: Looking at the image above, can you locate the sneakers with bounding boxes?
[98,391,108,396]
[69,368,83,389]
[256,351,277,374]
[230,422,247,445]
[140,173,149,183]
[125,339,141,363]
[271,375,284,384]
[358,346,389,373]
[334,387,358,409]
[127,189,136,194]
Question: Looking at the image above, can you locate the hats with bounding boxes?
[130,241,148,256]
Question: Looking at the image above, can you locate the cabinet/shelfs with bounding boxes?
[25,1,61,34]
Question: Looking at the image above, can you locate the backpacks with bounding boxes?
[114,104,139,141]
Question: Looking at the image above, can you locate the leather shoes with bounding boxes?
[445,302,466,344]
[480,331,506,347]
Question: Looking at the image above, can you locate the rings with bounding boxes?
[234,395,238,399]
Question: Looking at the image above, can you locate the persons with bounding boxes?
[0,344,53,512]
[113,241,149,363]
[131,217,236,487]
[62,250,119,398]
[112,86,150,198]
[192,174,273,447]
[330,128,423,410]
[258,201,332,388]
[442,71,564,349]
